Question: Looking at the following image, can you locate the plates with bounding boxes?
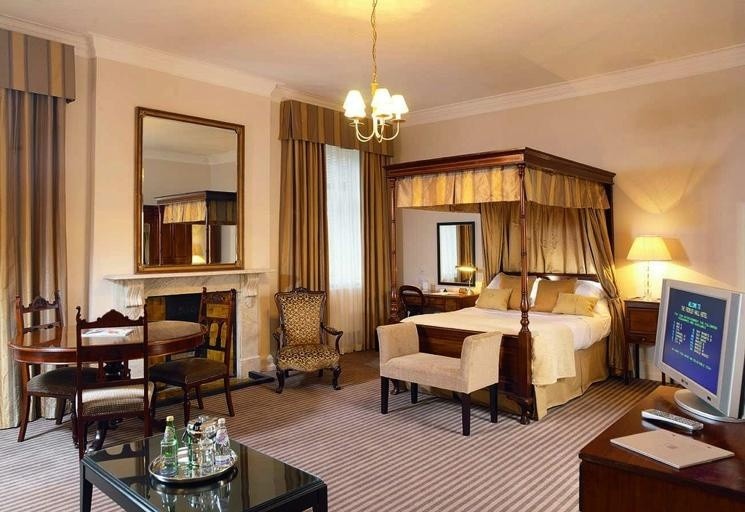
[148,446,239,485]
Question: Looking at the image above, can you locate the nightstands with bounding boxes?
[624,297,675,385]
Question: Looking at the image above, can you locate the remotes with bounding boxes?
[640,408,703,432]
[641,419,678,432]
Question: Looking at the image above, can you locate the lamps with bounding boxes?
[343,1,409,143]
[625,234,673,296]
[455,263,478,296]
[192,255,206,265]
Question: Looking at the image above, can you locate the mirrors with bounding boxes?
[134,106,245,273]
[437,222,475,286]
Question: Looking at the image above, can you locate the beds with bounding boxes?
[383,146,618,424]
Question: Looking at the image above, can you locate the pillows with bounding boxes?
[476,272,599,316]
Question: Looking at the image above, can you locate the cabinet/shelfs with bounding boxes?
[142,205,221,266]
[579,385,744,511]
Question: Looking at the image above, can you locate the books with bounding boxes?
[610,429,736,469]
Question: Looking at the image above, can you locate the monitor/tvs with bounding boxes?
[654,277,745,423]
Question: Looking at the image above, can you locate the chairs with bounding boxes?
[273,287,344,394]
[397,285,425,320]
[8,287,236,461]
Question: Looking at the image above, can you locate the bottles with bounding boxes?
[431,284,435,293]
[160,415,231,477]
[161,482,231,512]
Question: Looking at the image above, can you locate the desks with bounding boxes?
[403,293,479,319]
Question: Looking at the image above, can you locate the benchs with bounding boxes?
[376,322,503,436]
